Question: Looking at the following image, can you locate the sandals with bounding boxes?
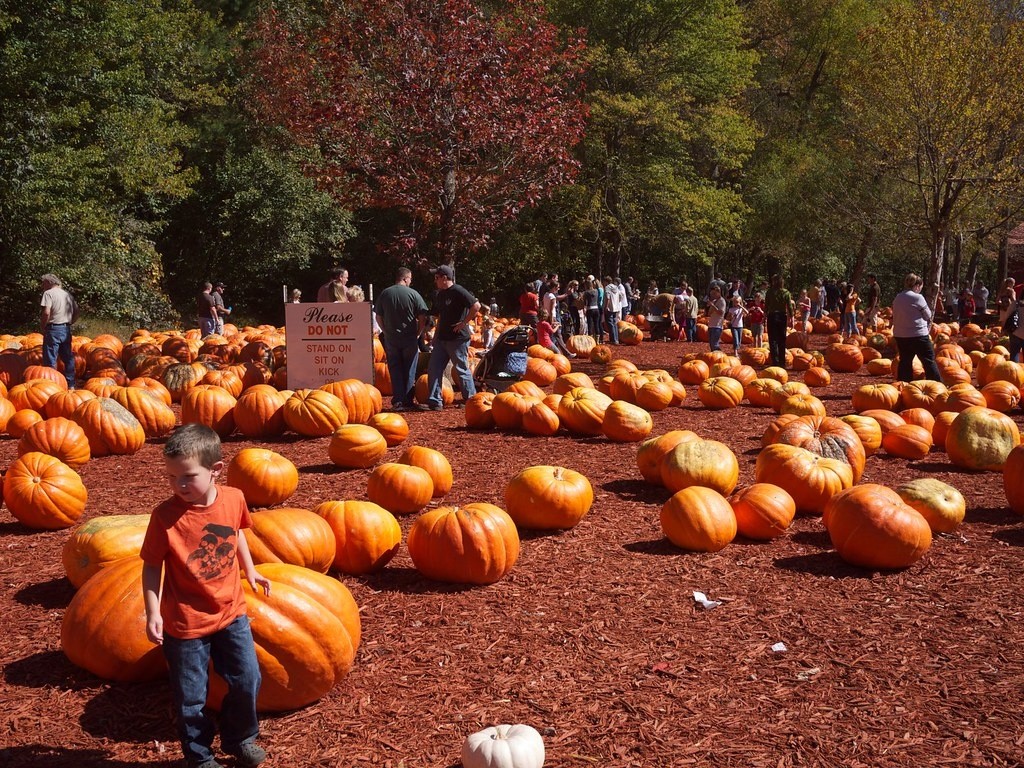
[222,739,266,765]
[187,757,220,768]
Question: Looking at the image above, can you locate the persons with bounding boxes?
[317,268,348,302]
[430,265,481,411]
[197,280,219,337]
[191,222,1024,418]
[764,274,795,370]
[705,285,726,353]
[861,274,886,337]
[212,282,232,335]
[726,295,749,360]
[845,284,860,339]
[518,282,540,345]
[375,267,429,411]
[603,276,623,345]
[139,424,275,768]
[287,288,301,303]
[38,273,81,390]
[892,273,944,383]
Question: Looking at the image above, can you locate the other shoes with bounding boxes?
[390,401,412,412]
[455,402,467,408]
[415,402,441,411]
[568,353,577,359]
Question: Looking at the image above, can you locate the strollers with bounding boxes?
[470,324,538,393]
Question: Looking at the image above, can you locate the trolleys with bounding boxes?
[645,312,672,342]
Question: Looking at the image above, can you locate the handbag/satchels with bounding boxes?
[1004,301,1019,335]
[504,353,528,374]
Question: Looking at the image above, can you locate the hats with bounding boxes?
[215,282,227,288]
[429,265,453,280]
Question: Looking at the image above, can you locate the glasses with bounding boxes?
[435,275,442,279]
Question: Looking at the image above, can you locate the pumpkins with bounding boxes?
[443,315,1024,569]
[0,324,521,717]
